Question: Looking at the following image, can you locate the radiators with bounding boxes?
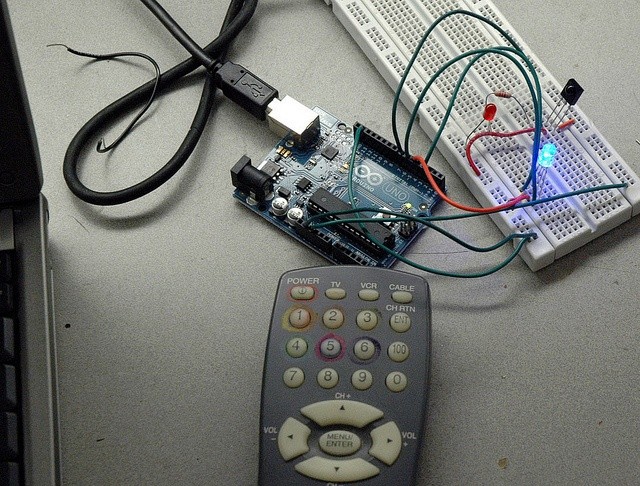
[258,265,430,486]
